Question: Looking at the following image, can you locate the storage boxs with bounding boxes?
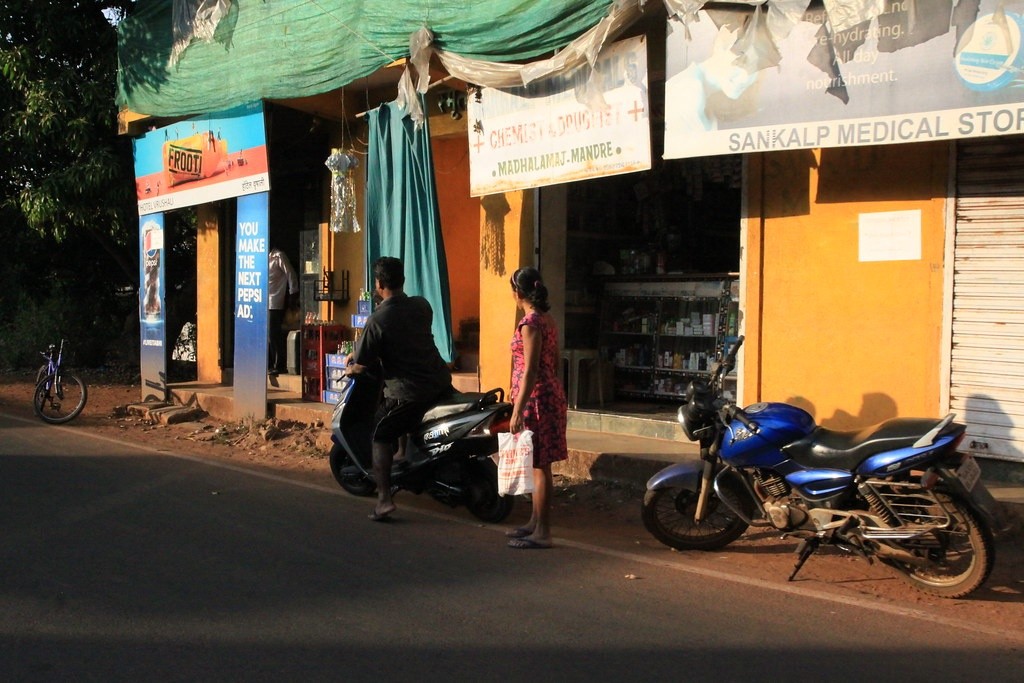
[302,300,371,405]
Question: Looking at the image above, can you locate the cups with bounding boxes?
[143,225,160,315]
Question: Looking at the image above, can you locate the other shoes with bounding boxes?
[271,369,288,374]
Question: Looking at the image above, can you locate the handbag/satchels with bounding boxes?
[497,430,534,497]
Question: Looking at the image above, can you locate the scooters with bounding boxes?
[329,355,517,525]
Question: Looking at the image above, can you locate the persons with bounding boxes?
[344,256,453,522]
[503,267,568,548]
[268,245,299,377]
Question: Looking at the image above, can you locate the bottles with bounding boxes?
[359,288,370,301]
[304,312,362,394]
[163,132,228,187]
[729,313,734,336]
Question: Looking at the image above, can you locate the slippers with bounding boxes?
[367,504,396,523]
[505,527,553,548]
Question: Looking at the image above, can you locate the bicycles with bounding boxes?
[34,338,88,425]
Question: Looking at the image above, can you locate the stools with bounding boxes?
[560,348,604,410]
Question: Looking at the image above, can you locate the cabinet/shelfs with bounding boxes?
[599,281,724,401]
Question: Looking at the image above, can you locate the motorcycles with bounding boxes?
[642,336,1002,599]
